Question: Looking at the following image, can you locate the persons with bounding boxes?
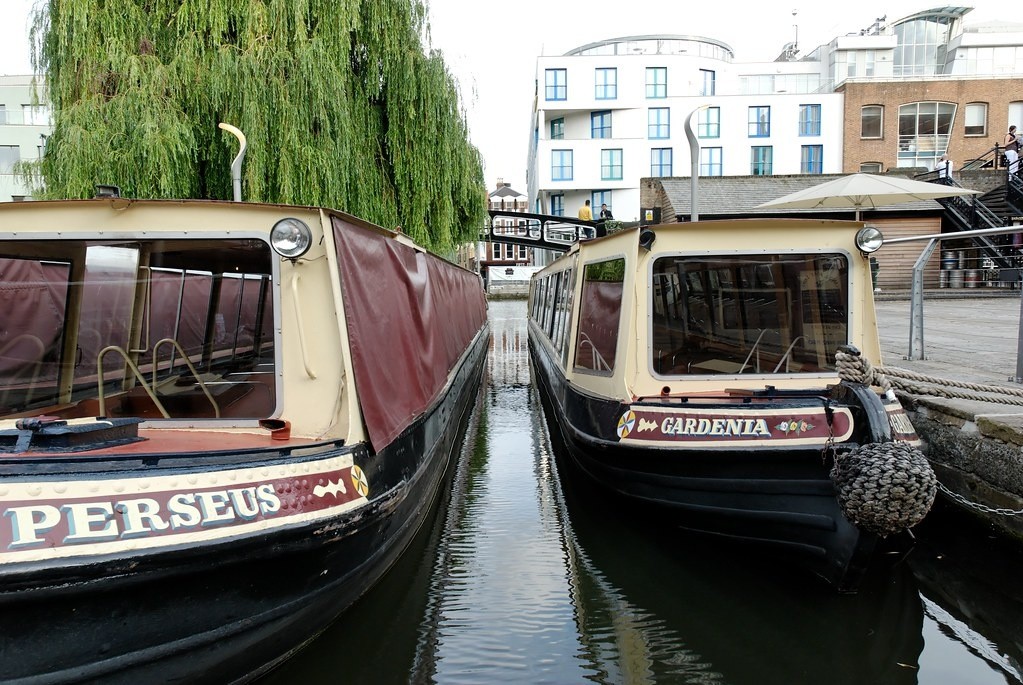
[1004,125,1020,181]
[600,203,613,217]
[935,153,953,185]
[579,199,593,238]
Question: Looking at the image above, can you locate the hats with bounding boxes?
[1009,125,1016,132]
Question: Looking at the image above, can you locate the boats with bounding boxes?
[0,184,492,685]
[526,206,927,532]
